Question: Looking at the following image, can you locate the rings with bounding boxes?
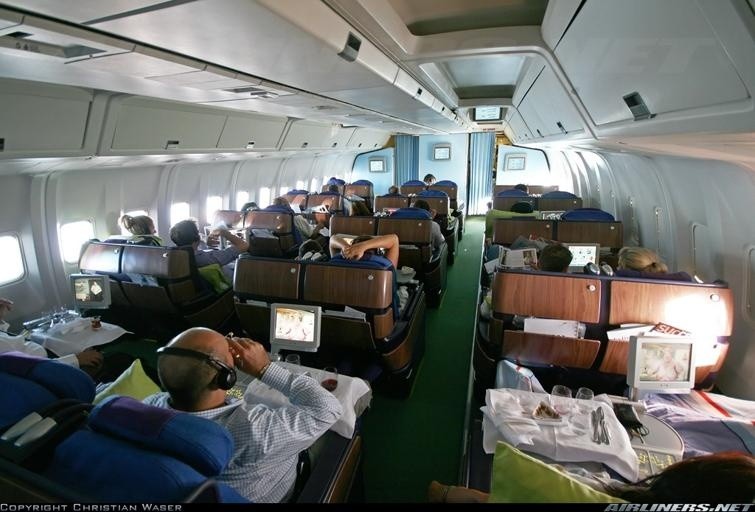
[234,353,240,360]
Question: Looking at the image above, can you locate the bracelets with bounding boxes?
[439,485,456,503]
[257,360,271,380]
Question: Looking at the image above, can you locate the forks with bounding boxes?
[591,405,613,446]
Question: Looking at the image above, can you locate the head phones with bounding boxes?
[351,236,385,256]
[155,346,238,389]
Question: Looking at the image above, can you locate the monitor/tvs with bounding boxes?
[504,153,527,172]
[223,227,250,250]
[368,157,387,174]
[541,210,567,222]
[626,333,696,394]
[563,242,601,272]
[69,274,111,310]
[204,225,223,251]
[432,143,453,161]
[382,206,400,217]
[473,107,503,121]
[267,303,322,352]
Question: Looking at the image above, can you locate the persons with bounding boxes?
[513,183,529,193]
[120,171,438,298]
[0,297,110,386]
[277,311,307,340]
[135,326,343,505]
[642,345,688,382]
[525,250,534,266]
[75,282,84,290]
[511,201,532,213]
[429,451,755,512]
[90,280,103,301]
[616,247,669,275]
[478,243,574,319]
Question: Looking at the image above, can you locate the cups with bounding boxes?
[5,297,17,313]
[89,315,102,330]
[284,354,304,377]
[549,384,572,416]
[320,365,340,391]
[266,349,281,365]
[575,386,594,416]
[36,300,83,329]
